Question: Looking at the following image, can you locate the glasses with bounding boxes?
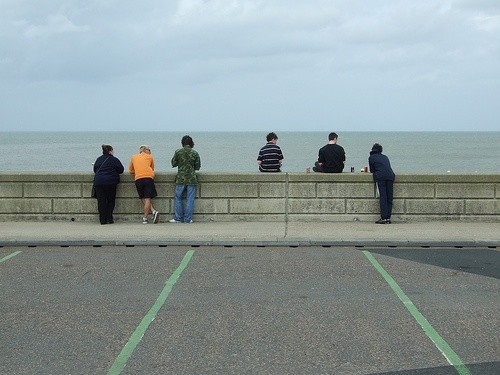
[146,149,150,152]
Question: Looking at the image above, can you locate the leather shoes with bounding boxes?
[375,218,391,224]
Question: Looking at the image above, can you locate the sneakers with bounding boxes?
[186,220,193,224]
[169,219,180,223]
[151,210,159,224]
[143,217,151,224]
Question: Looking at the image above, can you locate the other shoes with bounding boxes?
[101,221,114,225]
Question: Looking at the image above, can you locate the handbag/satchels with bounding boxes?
[91,180,96,199]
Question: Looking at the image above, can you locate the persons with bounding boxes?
[93,144,124,225]
[129,144,160,225]
[168,135,201,224]
[257,132,284,172]
[313,132,346,172]
[369,143,396,224]
